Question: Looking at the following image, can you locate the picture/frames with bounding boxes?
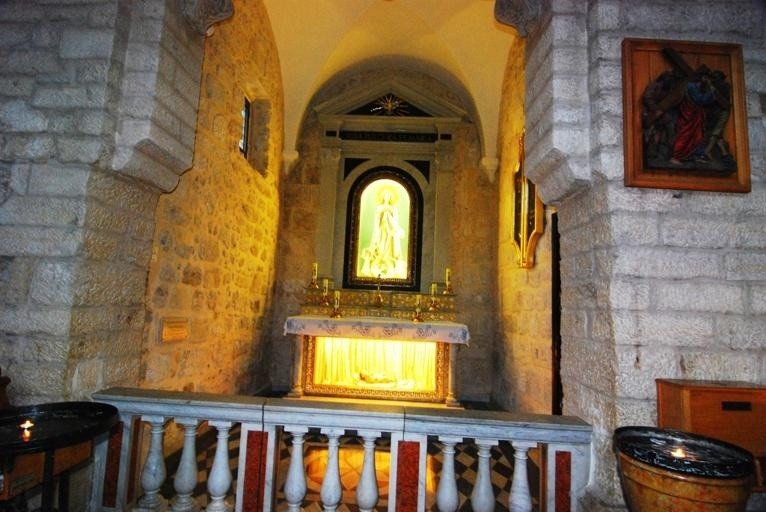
[620,35,752,195]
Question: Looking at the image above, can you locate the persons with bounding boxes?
[363,189,404,278]
[640,63,736,172]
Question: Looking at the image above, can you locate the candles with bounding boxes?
[310,263,340,308]
[415,267,451,313]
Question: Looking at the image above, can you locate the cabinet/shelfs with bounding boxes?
[653,376,766,493]
